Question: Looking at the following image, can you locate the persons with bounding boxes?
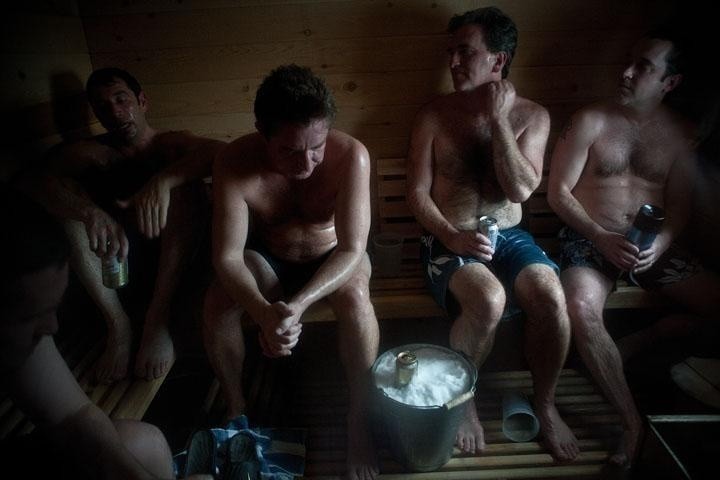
[5,72,231,385]
[197,65,384,478]
[403,5,583,467]
[545,15,716,476]
[2,193,174,480]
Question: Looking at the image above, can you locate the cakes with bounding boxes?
[478,216,499,254]
[395,350,418,389]
[101,242,130,290]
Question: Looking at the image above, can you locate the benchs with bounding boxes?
[0,159,719,480]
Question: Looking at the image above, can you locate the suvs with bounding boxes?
[369,343,477,473]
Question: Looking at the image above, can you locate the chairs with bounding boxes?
[226,431,261,480]
[180,429,218,480]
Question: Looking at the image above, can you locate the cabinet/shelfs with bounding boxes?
[624,203,665,263]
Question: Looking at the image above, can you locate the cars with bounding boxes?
[500,389,541,443]
[371,232,405,279]
